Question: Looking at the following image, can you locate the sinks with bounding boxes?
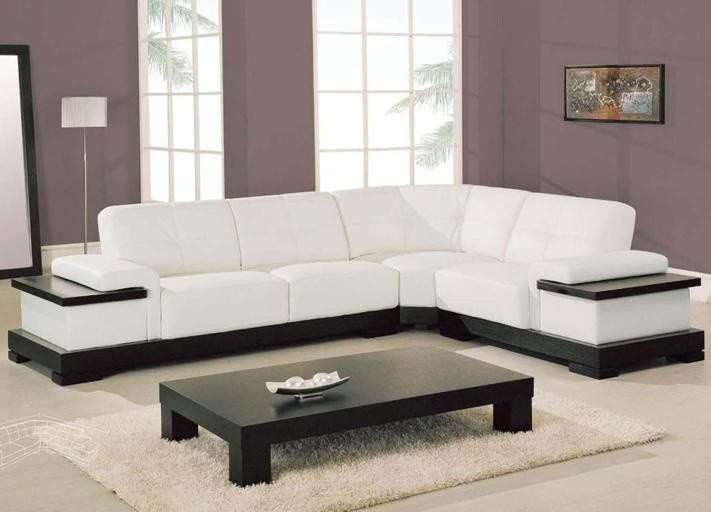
[7,182,704,386]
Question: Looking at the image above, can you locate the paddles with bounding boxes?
[34,367,665,512]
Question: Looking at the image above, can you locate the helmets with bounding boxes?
[266,370,351,402]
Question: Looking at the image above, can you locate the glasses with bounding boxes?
[60,96,108,253]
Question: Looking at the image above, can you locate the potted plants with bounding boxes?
[564,63,665,124]
